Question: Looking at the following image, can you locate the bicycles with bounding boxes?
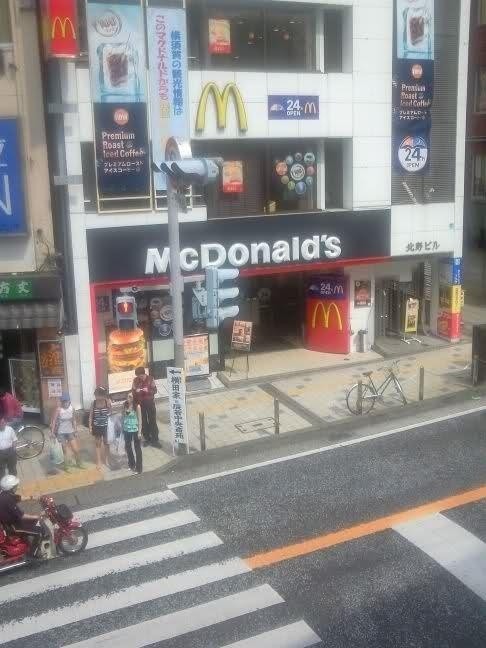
[1,422,44,459]
[346,360,407,415]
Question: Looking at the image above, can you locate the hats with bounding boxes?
[61,395,70,401]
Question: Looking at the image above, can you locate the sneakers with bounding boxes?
[31,556,38,559]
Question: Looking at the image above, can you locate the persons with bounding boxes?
[0,474,49,570]
[48,393,88,476]
[0,385,23,431]
[131,367,162,449]
[120,389,142,476]
[87,385,116,472]
[0,413,18,477]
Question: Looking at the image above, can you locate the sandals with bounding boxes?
[128,466,142,475]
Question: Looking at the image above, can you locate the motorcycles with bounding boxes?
[0,496,87,575]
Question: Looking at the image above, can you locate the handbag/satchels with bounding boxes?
[107,417,115,445]
[48,436,65,465]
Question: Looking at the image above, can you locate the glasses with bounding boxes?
[126,395,131,398]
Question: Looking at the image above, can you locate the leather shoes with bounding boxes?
[142,439,162,448]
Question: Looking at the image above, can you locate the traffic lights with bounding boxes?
[151,158,219,185]
[116,296,138,331]
[206,265,239,329]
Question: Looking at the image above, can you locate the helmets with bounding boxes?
[0,475,20,491]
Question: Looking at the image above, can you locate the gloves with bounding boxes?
[30,495,38,504]
[41,515,49,519]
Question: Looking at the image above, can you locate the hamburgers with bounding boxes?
[107,326,146,374]
